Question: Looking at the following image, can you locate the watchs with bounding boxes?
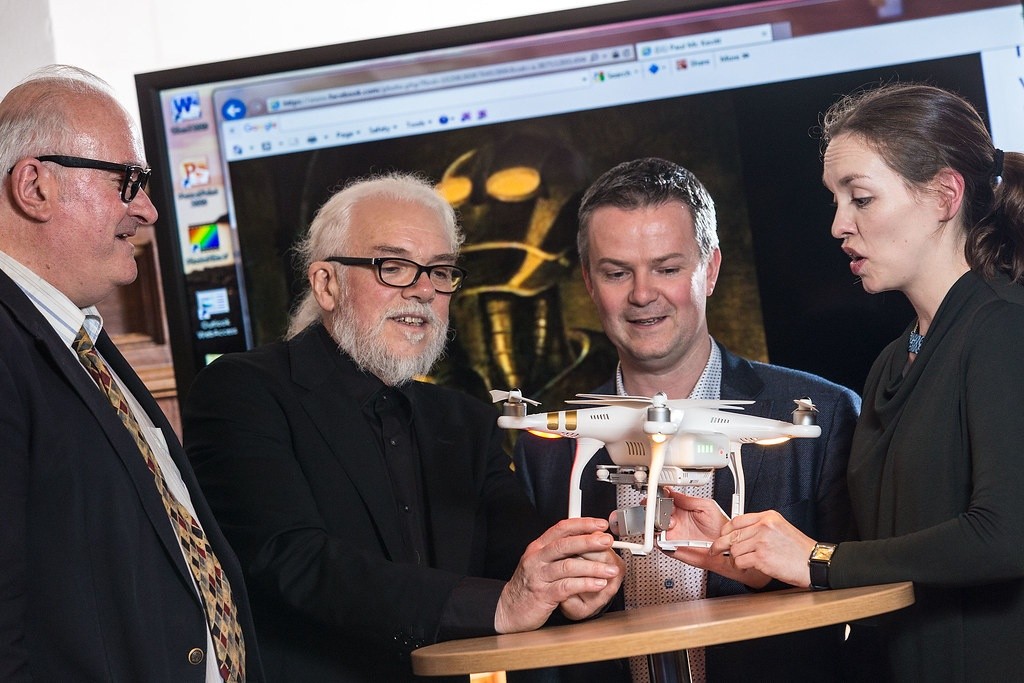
[807,542,838,589]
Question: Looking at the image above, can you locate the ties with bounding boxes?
[71,324,246,683]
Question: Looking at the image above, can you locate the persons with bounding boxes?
[182,175,625,683]
[514,157,863,683]
[0,77,263,683]
[641,86,1024,683]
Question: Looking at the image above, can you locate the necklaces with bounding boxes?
[907,320,924,354]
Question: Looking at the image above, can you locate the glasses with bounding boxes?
[7,154,152,203]
[324,257,468,294]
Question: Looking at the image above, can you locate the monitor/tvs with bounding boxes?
[125,0,1024,461]
